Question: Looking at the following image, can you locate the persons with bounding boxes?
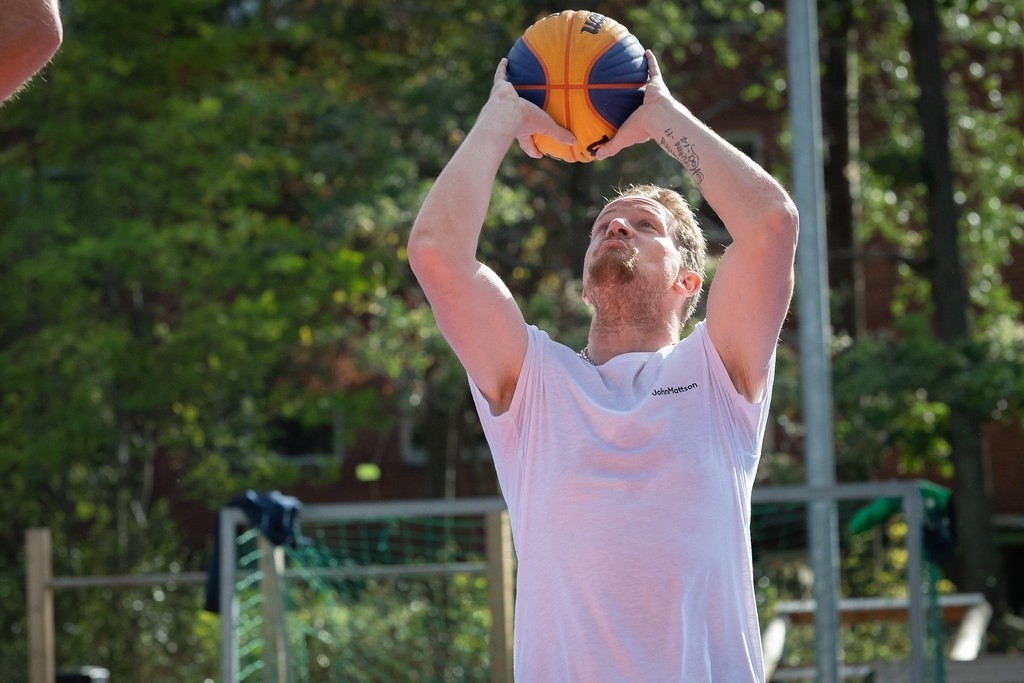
[0,0,63,107]
[408,48,800,683]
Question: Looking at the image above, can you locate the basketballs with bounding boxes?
[505,9,649,164]
